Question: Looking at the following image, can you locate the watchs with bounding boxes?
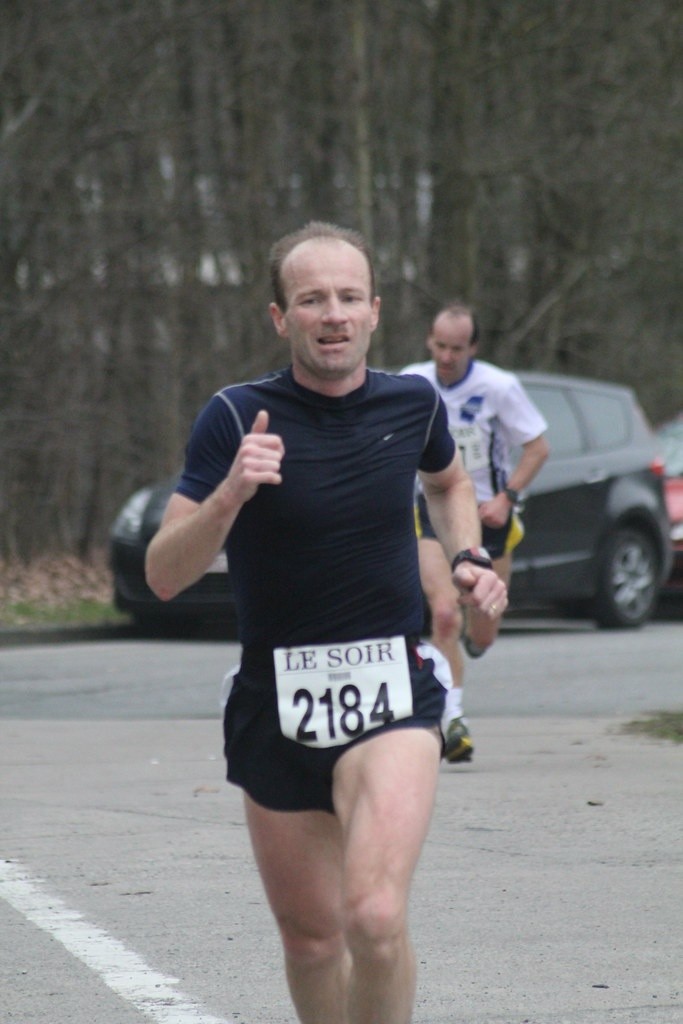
[451,548,492,572]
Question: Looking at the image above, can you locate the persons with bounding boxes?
[396,303,551,765]
[146,218,509,1024]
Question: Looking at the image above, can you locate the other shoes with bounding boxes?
[444,718,475,762]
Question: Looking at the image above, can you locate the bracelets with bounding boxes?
[503,489,518,503]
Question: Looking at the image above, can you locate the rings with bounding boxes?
[492,604,496,610]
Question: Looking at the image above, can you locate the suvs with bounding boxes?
[105,370,673,624]
